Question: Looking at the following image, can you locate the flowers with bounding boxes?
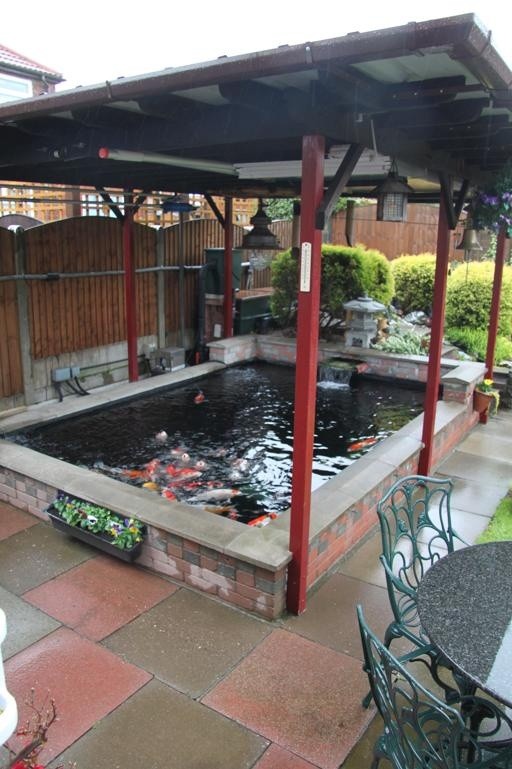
[464,167,512,238]
[476,378,500,415]
[52,492,144,549]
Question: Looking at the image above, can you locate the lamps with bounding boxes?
[235,198,286,274]
[367,158,417,223]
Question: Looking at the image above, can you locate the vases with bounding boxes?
[474,387,494,415]
